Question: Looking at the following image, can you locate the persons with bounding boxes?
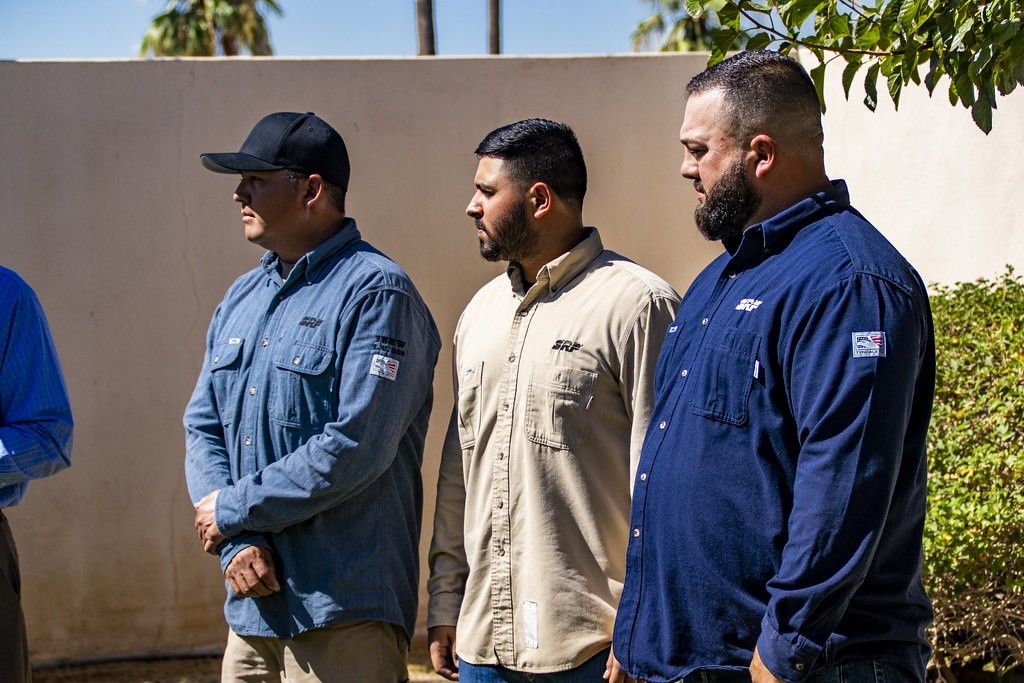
[180,112,443,682]
[0,266,75,682]
[424,118,683,683]
[613,49,936,682]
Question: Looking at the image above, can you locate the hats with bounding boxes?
[199,112,351,190]
